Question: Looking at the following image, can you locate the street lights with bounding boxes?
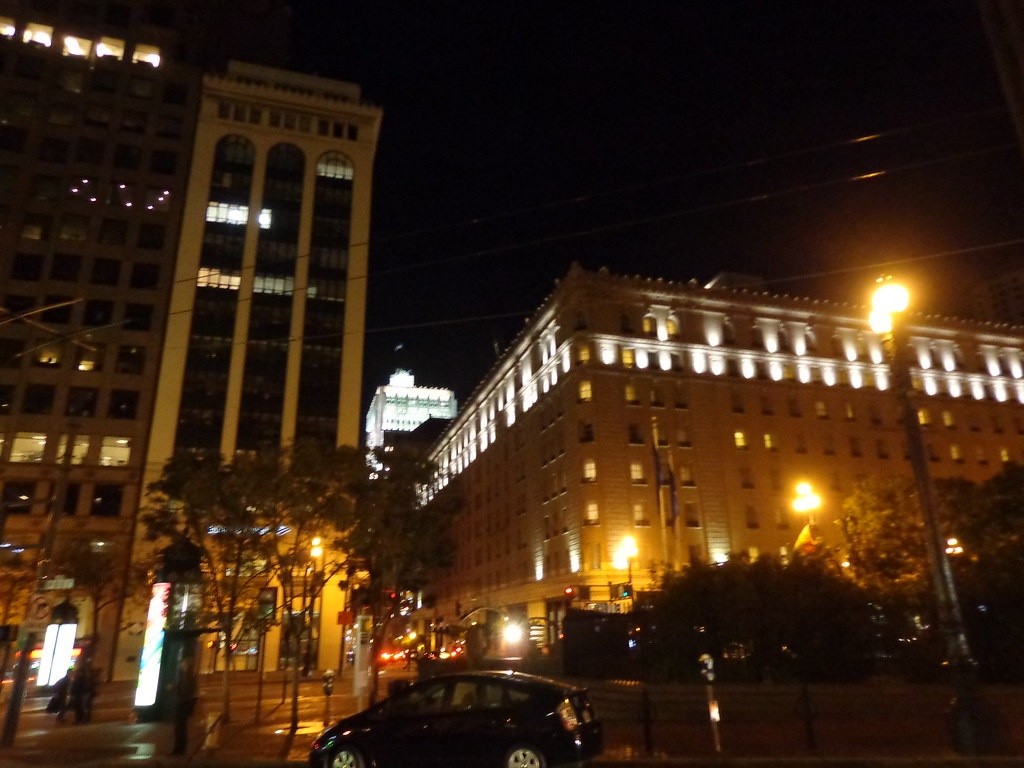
[868,260,1009,756]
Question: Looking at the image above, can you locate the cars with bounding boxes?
[308,670,603,768]
